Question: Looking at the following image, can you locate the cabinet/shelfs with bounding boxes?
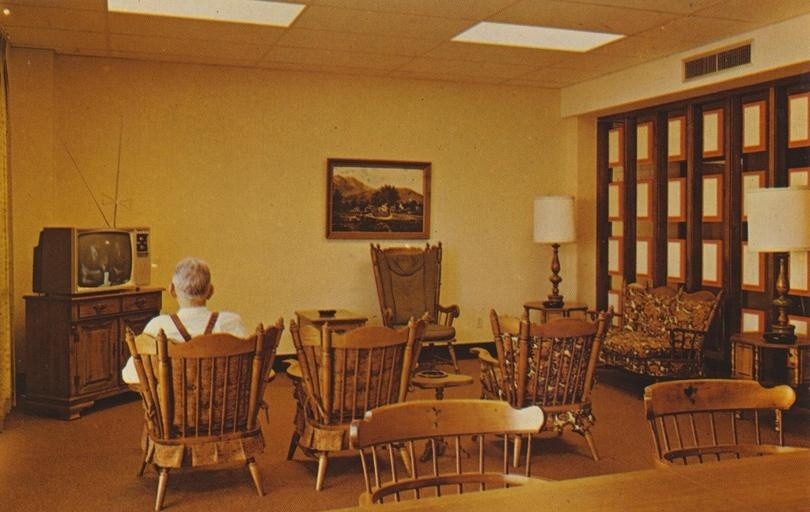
[19,285,168,422]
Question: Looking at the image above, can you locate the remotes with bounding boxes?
[318,309,336,315]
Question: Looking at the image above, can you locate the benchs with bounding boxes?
[587,282,729,384]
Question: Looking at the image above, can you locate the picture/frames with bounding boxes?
[323,157,432,243]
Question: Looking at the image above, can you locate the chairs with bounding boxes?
[367,241,465,376]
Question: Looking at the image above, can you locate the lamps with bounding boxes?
[746,185,810,344]
[530,191,577,309]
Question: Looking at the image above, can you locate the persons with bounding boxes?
[118,256,267,383]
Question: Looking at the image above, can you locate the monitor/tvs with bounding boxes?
[32,226,151,297]
